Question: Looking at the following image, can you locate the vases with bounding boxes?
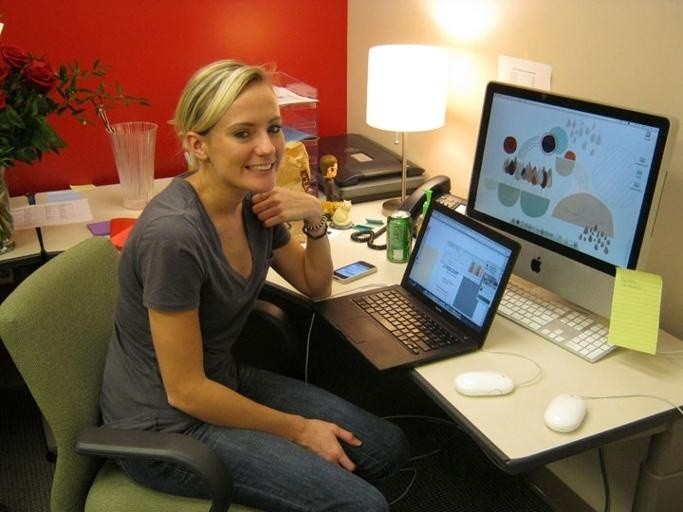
[0,181,16,258]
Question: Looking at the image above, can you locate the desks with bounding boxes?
[1,176,320,300]
[324,201,682,474]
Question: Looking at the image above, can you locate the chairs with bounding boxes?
[0,234,292,504]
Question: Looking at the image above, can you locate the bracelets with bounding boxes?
[301,223,328,239]
[302,215,327,231]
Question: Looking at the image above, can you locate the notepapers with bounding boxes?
[87,222,111,236]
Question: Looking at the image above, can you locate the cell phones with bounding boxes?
[333,261,377,283]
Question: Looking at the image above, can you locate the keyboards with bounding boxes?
[495,282,620,362]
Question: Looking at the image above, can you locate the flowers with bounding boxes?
[0,47,155,173]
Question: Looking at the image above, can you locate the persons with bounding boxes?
[319,153,340,204]
[97,58,414,511]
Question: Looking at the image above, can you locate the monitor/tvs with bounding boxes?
[465,80,680,319]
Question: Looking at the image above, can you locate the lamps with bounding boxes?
[364,42,446,215]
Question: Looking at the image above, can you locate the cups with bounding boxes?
[103,121,159,211]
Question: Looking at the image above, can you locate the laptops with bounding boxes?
[312,200,522,375]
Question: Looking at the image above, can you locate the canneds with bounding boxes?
[386,210,413,264]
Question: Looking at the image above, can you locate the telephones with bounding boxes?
[398,175,467,239]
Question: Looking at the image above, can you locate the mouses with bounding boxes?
[545,394,587,432]
[456,372,516,395]
